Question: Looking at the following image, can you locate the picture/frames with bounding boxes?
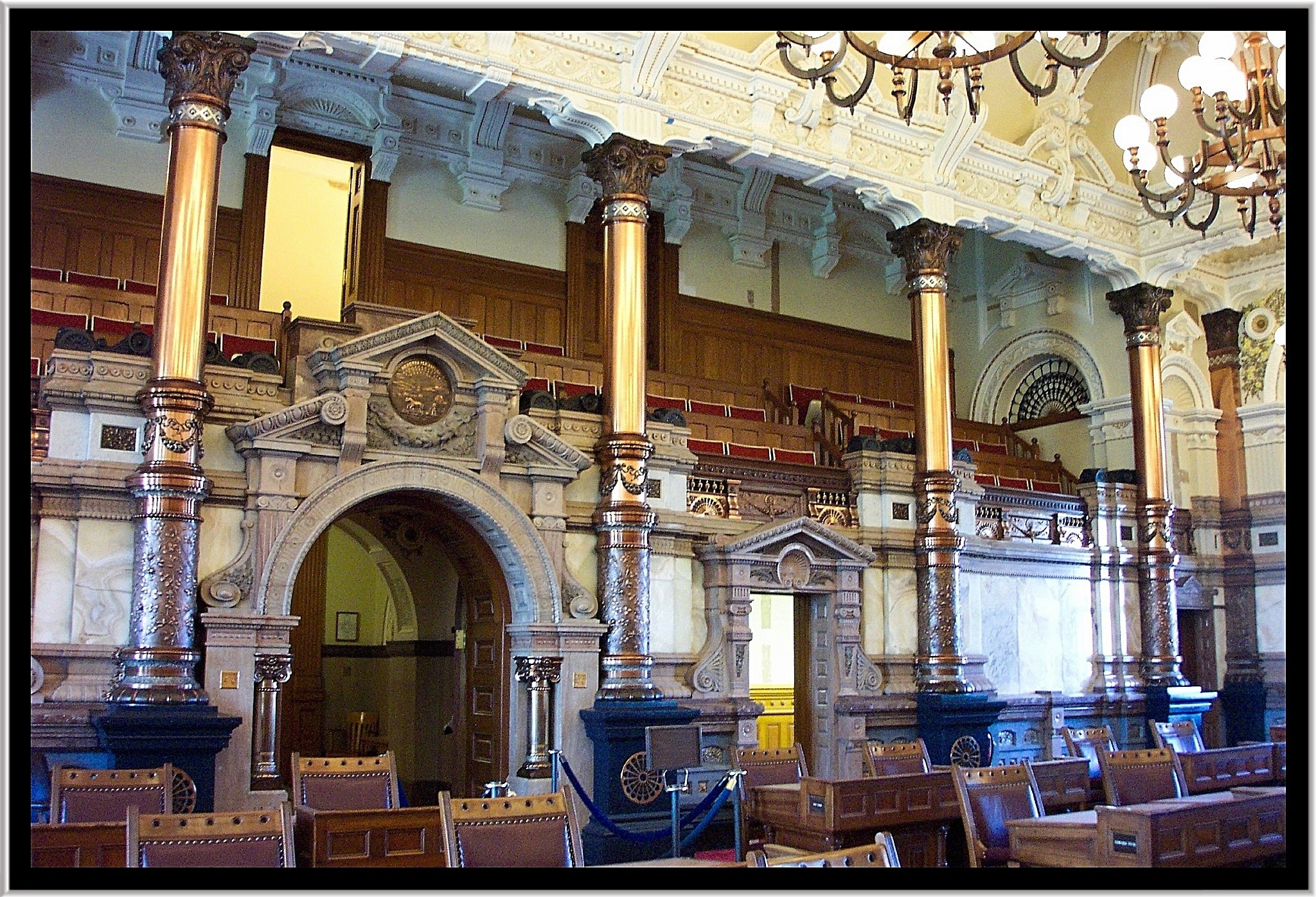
[336,612,359,642]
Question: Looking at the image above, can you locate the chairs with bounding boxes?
[31,267,1062,499]
[31,712,1287,869]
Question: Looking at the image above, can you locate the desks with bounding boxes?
[1005,787,1287,868]
[749,772,960,868]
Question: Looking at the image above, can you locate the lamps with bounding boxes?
[774,31,1285,250]
